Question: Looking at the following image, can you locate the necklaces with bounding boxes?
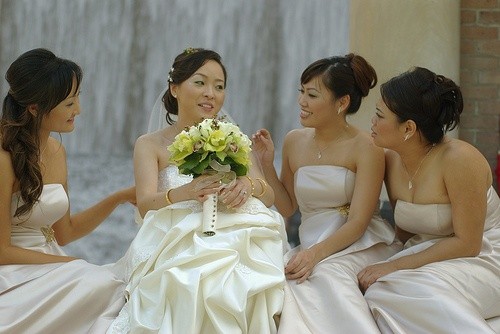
[38,144,48,172]
[401,142,434,189]
[314,125,349,159]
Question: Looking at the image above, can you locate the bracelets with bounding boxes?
[166,187,175,206]
[250,177,255,198]
[257,178,268,199]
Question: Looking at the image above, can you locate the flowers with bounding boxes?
[165,112,253,236]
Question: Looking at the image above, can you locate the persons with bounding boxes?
[250,53,404,334]
[0,50,136,334]
[358,67,500,334]
[109,49,280,334]
